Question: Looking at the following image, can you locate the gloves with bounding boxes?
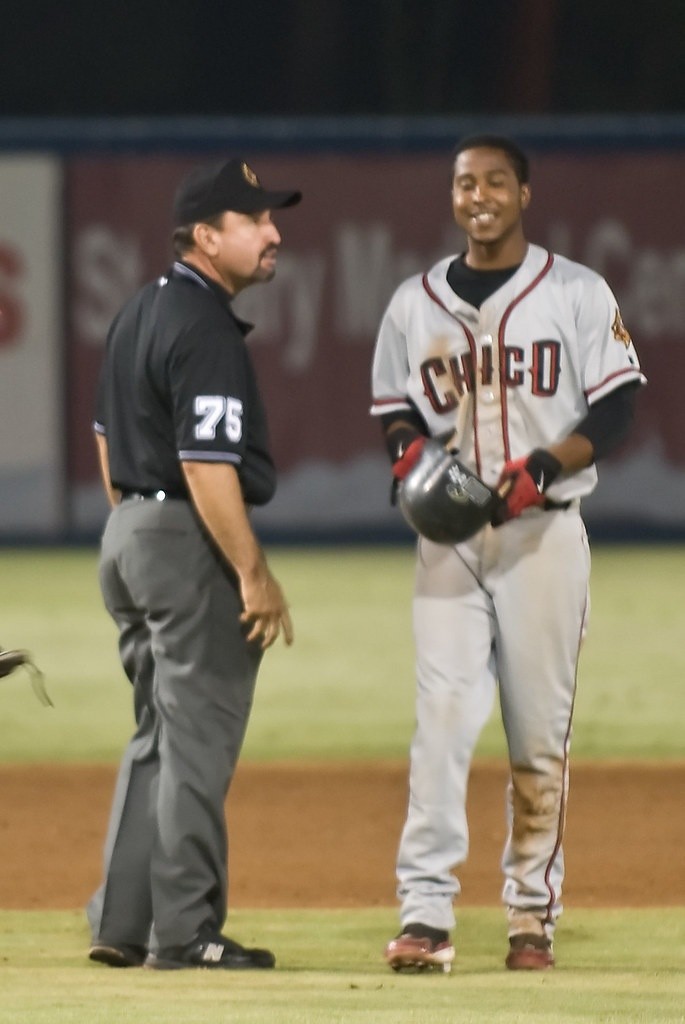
[385,423,461,481]
[490,446,576,528]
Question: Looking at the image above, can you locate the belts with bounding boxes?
[120,488,192,501]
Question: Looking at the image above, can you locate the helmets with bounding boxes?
[396,439,505,546]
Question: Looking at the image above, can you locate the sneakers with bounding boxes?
[151,933,276,970]
[384,922,456,973]
[505,932,554,970]
[88,943,148,967]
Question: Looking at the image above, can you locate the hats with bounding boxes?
[174,156,303,223]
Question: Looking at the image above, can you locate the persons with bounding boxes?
[368,132,645,977]
[91,162,301,968]
